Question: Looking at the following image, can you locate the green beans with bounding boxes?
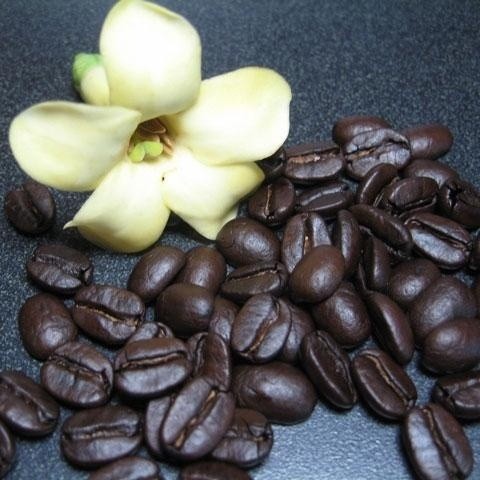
[0,116,480,480]
